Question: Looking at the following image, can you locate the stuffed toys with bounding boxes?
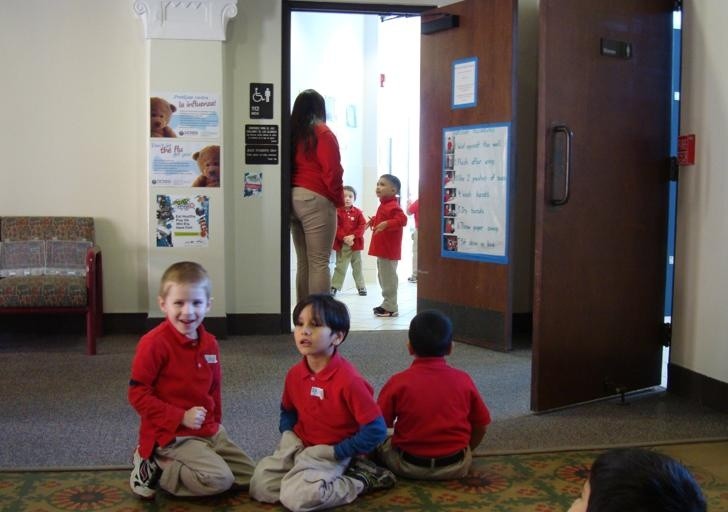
[150,97,178,138]
[192,145,220,187]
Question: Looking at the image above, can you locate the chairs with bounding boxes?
[0,214,104,355]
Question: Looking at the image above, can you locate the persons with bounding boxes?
[568,446,711,512]
[405,196,419,281]
[374,310,491,481]
[443,132,456,252]
[364,173,407,316]
[126,262,256,498]
[248,293,396,511]
[290,89,345,303]
[330,188,367,297]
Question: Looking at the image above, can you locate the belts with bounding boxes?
[396,447,463,468]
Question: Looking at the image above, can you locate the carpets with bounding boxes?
[0,432,728,511]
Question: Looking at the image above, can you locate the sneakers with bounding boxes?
[373,307,383,312]
[348,455,396,489]
[330,287,335,296]
[373,310,397,317]
[358,288,366,295]
[129,445,163,501]
